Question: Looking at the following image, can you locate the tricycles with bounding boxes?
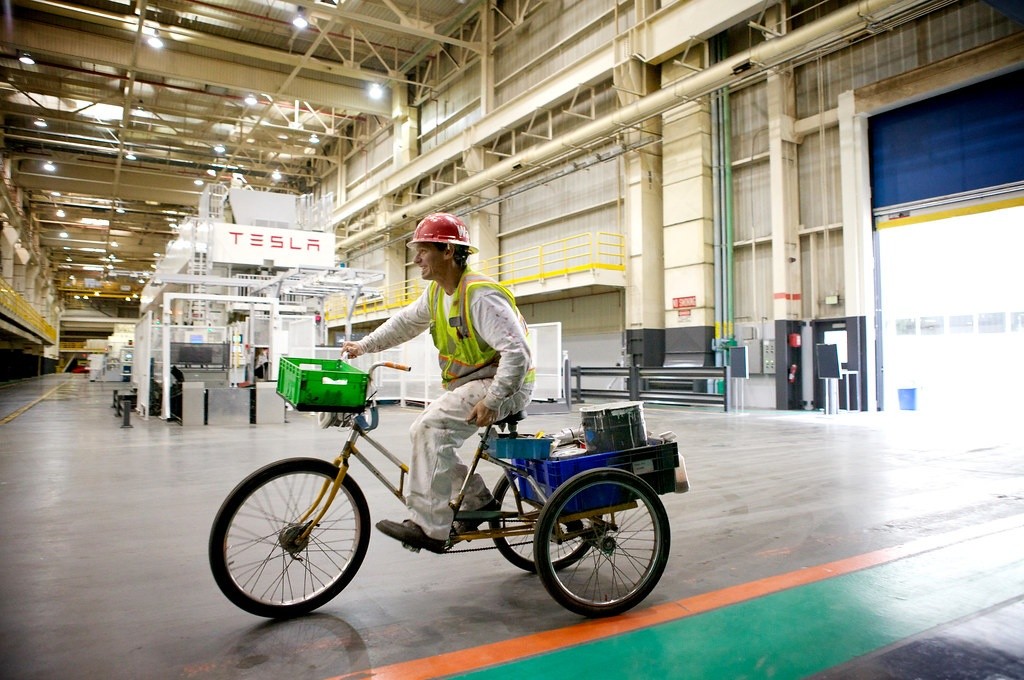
[209,352,680,618]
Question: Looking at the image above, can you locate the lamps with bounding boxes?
[18,51,35,65]
[292,6,308,28]
[33,118,47,126]
[148,29,163,49]
[44,160,56,171]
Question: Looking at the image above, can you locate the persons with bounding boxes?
[341,212,537,555]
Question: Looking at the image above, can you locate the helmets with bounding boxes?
[405,213,479,254]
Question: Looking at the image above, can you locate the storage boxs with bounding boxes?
[276,357,369,413]
[511,433,679,514]
[489,438,555,460]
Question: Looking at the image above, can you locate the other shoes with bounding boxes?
[450,499,501,538]
[376,519,445,554]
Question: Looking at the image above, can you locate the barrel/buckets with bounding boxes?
[580,401,647,455]
[897,388,918,412]
